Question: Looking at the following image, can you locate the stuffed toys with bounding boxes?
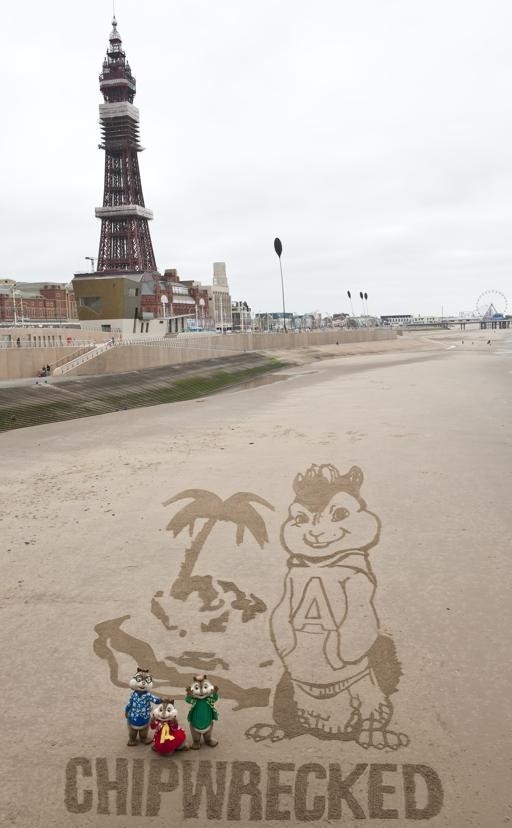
[150,700,190,755]
[123,669,164,746]
[185,675,220,748]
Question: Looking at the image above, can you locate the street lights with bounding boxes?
[271,238,289,332]
[345,290,355,317]
[359,291,365,316]
[364,292,369,318]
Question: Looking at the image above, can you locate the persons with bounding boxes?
[39,364,50,376]
[112,335,114,344]
[17,337,20,347]
